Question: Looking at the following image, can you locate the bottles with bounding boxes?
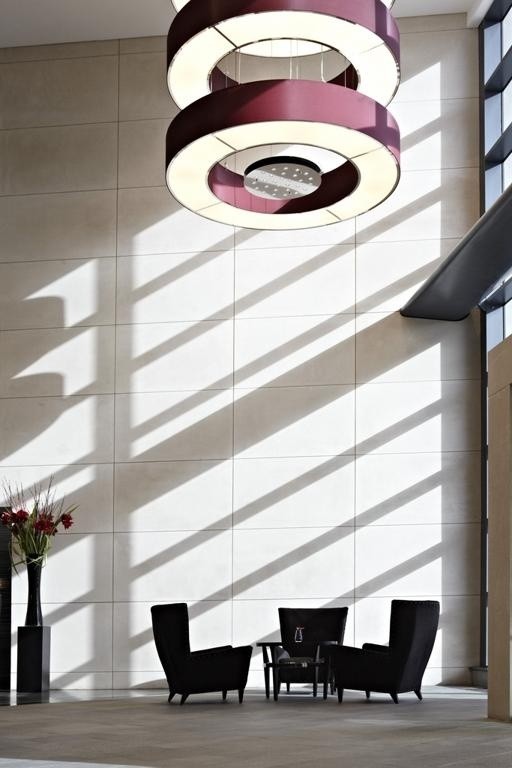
[295,625,305,642]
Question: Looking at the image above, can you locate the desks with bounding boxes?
[256,640,338,700]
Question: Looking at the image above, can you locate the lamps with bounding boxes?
[163,0,402,232]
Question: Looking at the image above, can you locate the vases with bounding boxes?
[24,553,44,627]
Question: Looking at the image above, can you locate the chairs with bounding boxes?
[270,606,349,699]
[330,599,440,704]
[150,602,255,706]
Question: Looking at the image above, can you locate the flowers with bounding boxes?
[0,471,81,577]
